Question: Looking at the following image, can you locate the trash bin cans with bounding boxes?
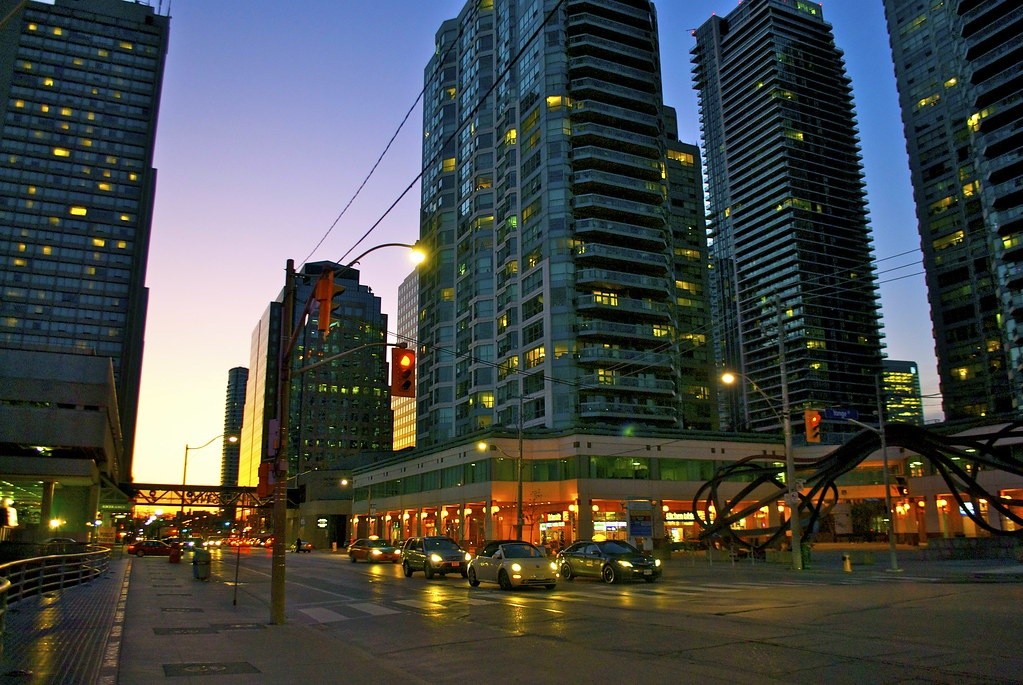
[169,542,180,563]
[192,550,211,580]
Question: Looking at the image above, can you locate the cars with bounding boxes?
[127,540,184,557]
[222,537,262,548]
[555,540,664,584]
[38,537,89,555]
[265,538,275,548]
[290,541,312,553]
[467,540,557,591]
[346,535,402,563]
[168,537,184,548]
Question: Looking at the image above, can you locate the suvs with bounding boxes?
[207,536,223,549]
[401,535,470,580]
[183,537,205,551]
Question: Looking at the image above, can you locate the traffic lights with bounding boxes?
[804,409,821,443]
[897,476,910,496]
[316,269,347,337]
[391,347,417,398]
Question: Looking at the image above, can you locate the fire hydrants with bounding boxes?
[843,554,853,573]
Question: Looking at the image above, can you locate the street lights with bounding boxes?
[176,435,237,558]
[268,241,432,611]
[477,442,524,542]
[721,372,804,571]
[50,519,59,539]
